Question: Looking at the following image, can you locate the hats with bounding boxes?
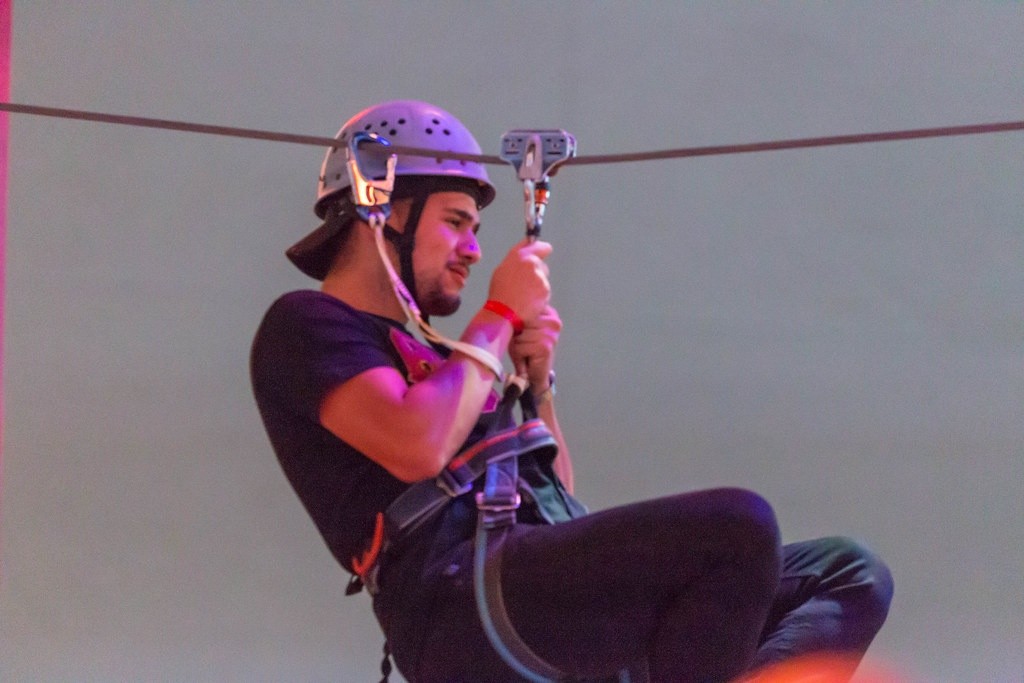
[285,175,485,281]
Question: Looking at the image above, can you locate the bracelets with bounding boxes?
[518,371,557,406]
[483,300,524,332]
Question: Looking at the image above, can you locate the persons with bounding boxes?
[249,99,893,683]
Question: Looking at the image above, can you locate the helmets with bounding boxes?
[314,99,496,220]
